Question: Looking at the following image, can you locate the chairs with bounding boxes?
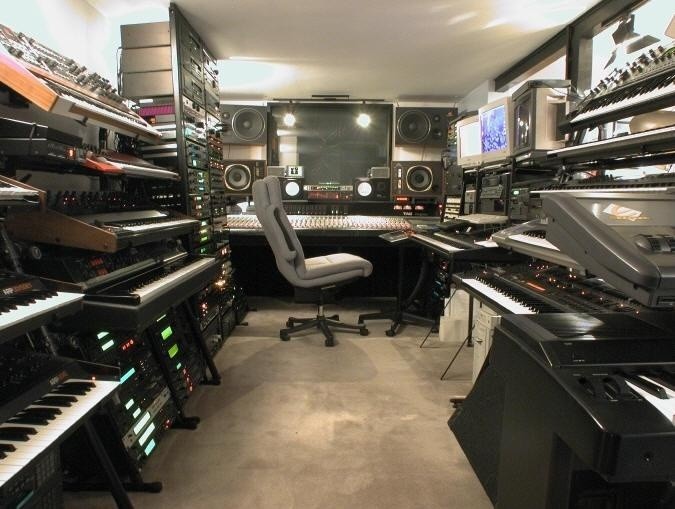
[252,174,373,347]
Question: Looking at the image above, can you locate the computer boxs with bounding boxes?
[470,308,502,387]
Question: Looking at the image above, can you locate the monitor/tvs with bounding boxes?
[456,85,568,173]
[263,100,396,185]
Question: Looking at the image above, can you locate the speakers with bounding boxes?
[353,178,390,201]
[266,166,285,177]
[393,106,449,148]
[390,160,445,197]
[278,178,305,200]
[219,104,268,145]
[371,167,390,179]
[222,159,265,194]
